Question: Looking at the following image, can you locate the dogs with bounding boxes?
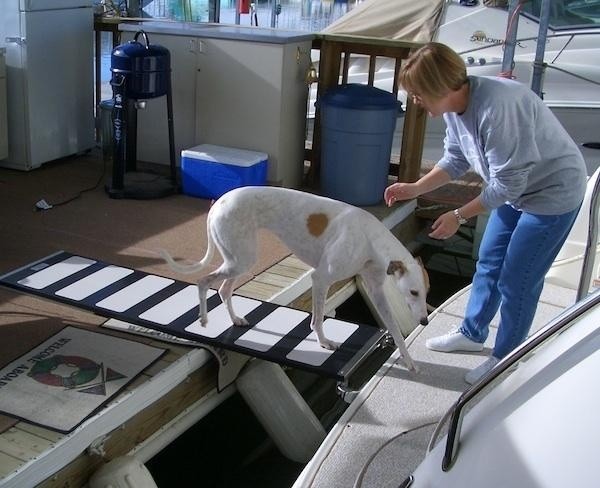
[157,185,432,375]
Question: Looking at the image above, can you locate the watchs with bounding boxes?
[454,210,468,225]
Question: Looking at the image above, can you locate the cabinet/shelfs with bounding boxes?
[118,20,316,190]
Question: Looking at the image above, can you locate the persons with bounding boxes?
[387,42,587,383]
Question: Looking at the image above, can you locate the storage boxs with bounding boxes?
[180,143,269,200]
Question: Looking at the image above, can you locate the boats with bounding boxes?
[290,166,599,486]
[306,1,599,174]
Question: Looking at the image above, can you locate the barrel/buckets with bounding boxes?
[314,83,405,206]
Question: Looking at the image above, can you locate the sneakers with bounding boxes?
[426,331,483,353]
[464,356,499,385]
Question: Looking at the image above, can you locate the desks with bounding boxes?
[307,31,428,182]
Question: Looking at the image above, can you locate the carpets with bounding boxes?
[0,318,254,436]
